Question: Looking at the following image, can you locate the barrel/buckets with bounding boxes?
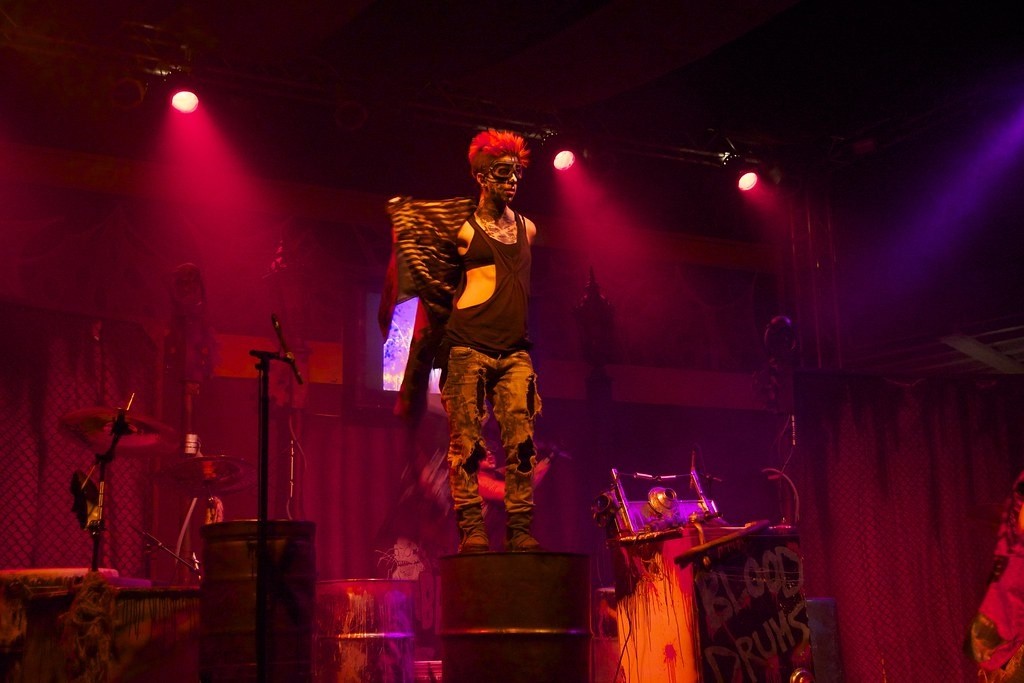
[439,549,593,683]
[199,519,313,683]
[315,578,417,683]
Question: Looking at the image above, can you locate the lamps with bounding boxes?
[723,157,757,191]
[541,135,575,171]
[169,67,198,113]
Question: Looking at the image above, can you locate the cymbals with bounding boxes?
[147,454,259,499]
[54,406,179,459]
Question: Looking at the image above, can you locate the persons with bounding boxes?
[391,130,541,553]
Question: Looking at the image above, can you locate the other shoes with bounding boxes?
[505,535,539,550]
[464,530,488,551]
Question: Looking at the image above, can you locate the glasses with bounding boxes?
[487,162,524,179]
